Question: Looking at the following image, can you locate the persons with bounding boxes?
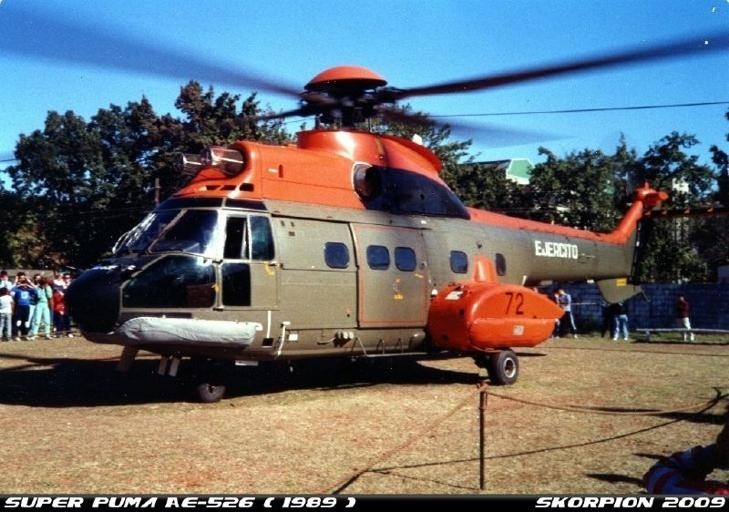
[0,267,74,340]
[601,299,613,339]
[612,303,628,340]
[531,285,577,340]
[644,425,729,497]
[677,294,695,341]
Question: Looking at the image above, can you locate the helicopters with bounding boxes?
[0,2,728,412]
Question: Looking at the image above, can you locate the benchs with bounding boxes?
[635,328,729,342]
